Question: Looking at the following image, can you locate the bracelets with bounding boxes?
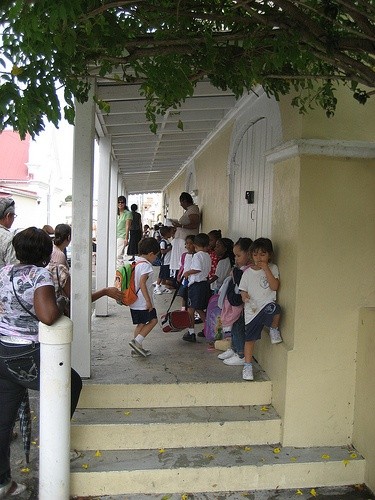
[181,224,184,229]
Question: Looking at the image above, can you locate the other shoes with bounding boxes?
[161,287,172,293]
[130,349,151,357]
[152,281,161,287]
[194,318,202,323]
[183,331,197,343]
[71,449,83,461]
[198,329,204,337]
[11,433,17,439]
[129,339,147,358]
[0,481,26,498]
[153,288,162,295]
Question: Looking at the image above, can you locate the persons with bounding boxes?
[177,230,282,380]
[172,192,199,239]
[127,204,143,261]
[117,196,133,269]
[49,224,126,304]
[128,237,160,357]
[143,222,176,295]
[0,196,83,498]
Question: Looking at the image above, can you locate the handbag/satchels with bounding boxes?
[161,311,191,333]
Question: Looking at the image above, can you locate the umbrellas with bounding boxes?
[17,387,30,463]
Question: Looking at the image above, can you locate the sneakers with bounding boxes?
[243,367,254,380]
[223,354,245,365]
[217,349,235,359]
[270,328,282,344]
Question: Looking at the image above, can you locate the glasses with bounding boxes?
[11,214,18,218]
[118,201,124,204]
[3,201,15,214]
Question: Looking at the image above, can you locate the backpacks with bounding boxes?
[150,240,167,266]
[114,262,150,307]
